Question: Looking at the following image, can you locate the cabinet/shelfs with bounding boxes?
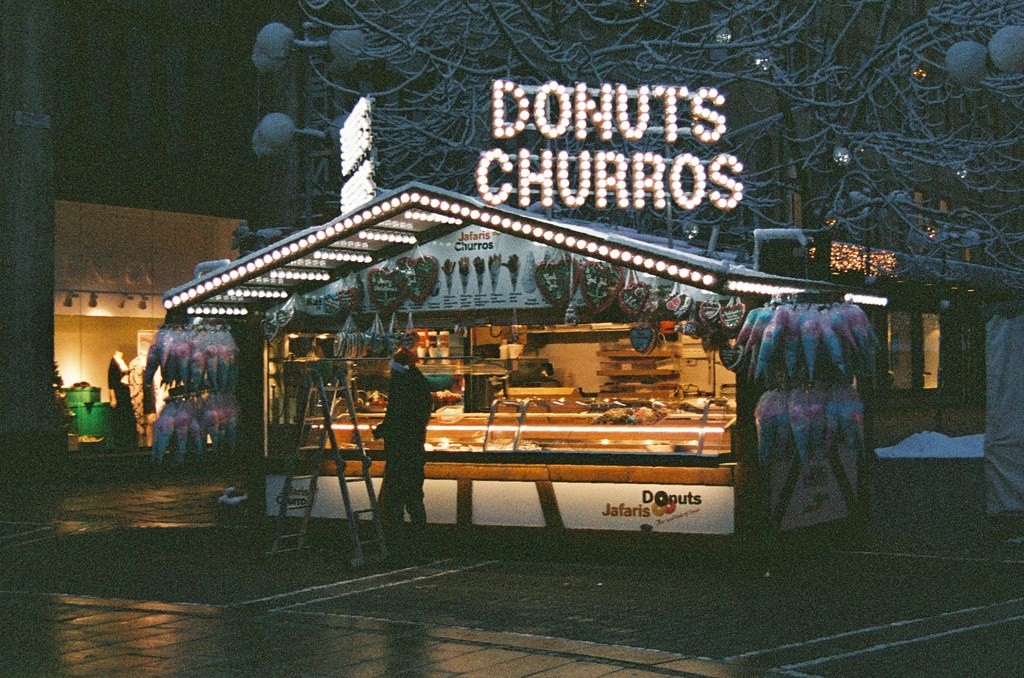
[597,350,682,400]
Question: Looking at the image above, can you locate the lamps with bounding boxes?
[118,301,126,308]
[138,301,146,310]
[63,292,73,307]
[88,293,98,307]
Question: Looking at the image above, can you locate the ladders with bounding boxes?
[257,357,394,577]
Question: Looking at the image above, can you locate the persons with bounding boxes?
[107,348,157,451]
[372,348,427,540]
